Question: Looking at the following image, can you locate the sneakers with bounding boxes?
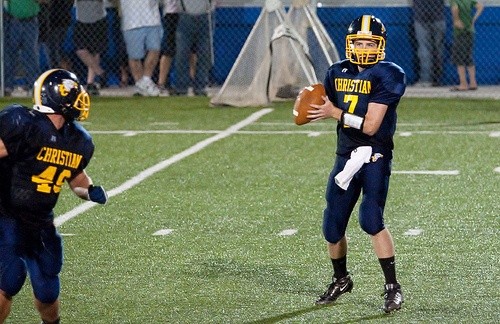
[316,275,353,306]
[382,282,403,313]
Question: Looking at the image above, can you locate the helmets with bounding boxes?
[32,69,90,120]
[346,15,388,64]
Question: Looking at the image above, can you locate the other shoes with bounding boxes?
[158,84,177,95]
[187,84,198,95]
[133,76,160,97]
[84,82,99,94]
[467,86,477,91]
[450,86,468,91]
[97,70,108,89]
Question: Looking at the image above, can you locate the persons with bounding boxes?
[0,0,71,96]
[306,14,406,313]
[411,0,447,88]
[451,0,484,90]
[118,0,163,97]
[169,0,211,96]
[158,0,198,96]
[0,69,106,324]
[73,0,110,96]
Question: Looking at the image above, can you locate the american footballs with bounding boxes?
[293,83,326,126]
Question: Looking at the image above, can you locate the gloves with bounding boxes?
[88,184,107,204]
